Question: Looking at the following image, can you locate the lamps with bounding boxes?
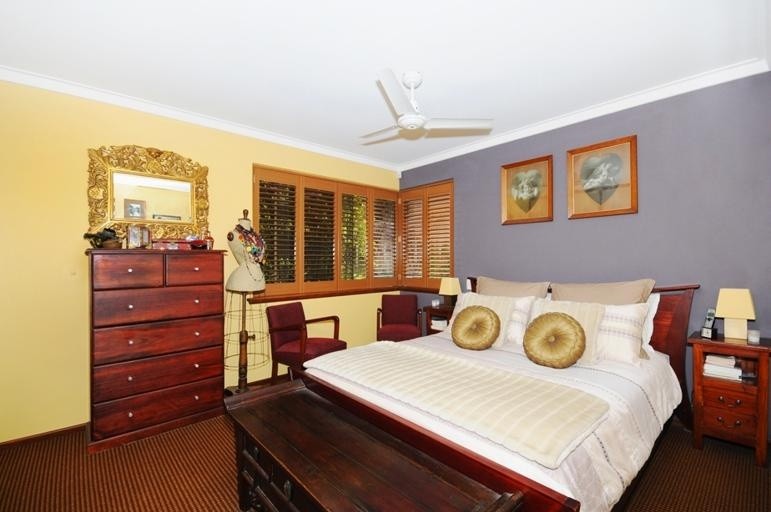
[438,277,460,310]
[715,288,757,341]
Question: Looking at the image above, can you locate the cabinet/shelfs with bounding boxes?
[220,377,580,511]
[85,247,225,441]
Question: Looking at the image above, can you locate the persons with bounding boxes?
[128,205,141,217]
[226,219,266,396]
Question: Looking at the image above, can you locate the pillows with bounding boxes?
[551,278,655,358]
[527,299,648,368]
[473,276,550,301]
[449,291,530,353]
[524,312,585,369]
[645,293,661,354]
[451,305,501,350]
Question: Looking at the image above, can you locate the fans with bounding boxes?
[356,73,496,147]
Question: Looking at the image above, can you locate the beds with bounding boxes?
[303,280,701,511]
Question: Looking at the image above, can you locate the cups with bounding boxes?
[747,329,760,344]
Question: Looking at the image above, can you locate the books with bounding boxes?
[702,355,742,383]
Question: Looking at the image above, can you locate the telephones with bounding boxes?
[700,308,718,340]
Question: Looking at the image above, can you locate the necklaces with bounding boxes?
[234,225,266,282]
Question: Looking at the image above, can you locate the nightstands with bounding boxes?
[686,330,771,466]
[422,303,454,336]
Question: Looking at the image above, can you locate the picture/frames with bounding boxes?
[500,154,554,225]
[565,135,638,219]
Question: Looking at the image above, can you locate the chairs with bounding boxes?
[375,294,423,342]
[266,301,347,377]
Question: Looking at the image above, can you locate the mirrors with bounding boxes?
[86,144,211,244]
